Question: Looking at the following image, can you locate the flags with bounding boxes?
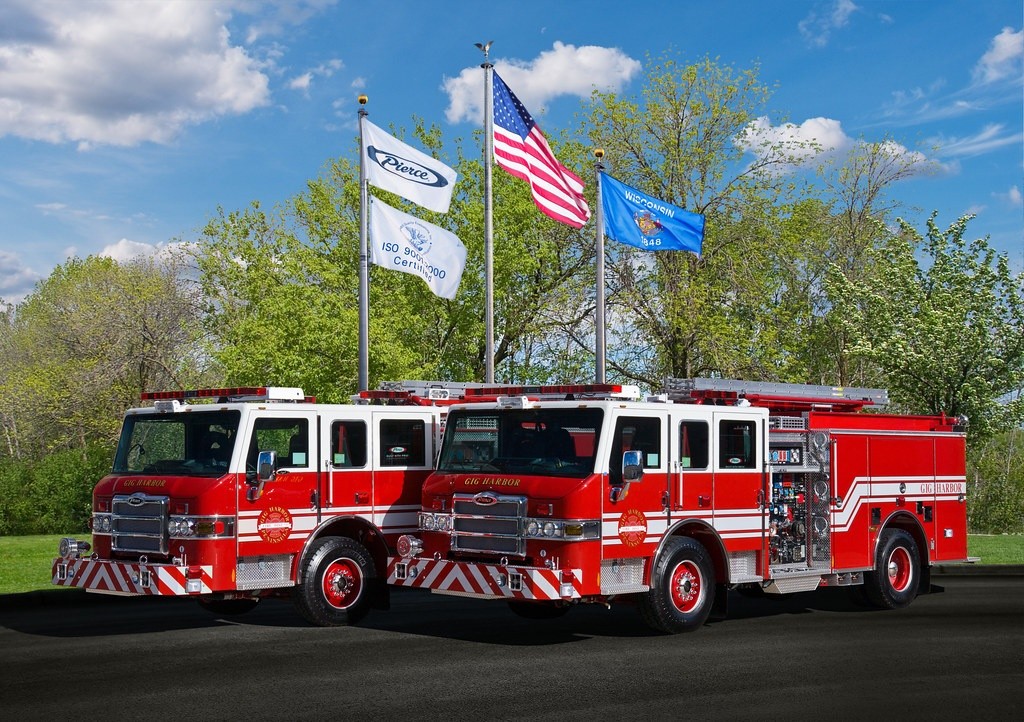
[486,62,591,230]
[363,193,468,300]
[359,114,457,213]
[597,171,706,261]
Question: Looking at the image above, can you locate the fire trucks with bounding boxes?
[386,377,968,634]
[52,381,635,627]
